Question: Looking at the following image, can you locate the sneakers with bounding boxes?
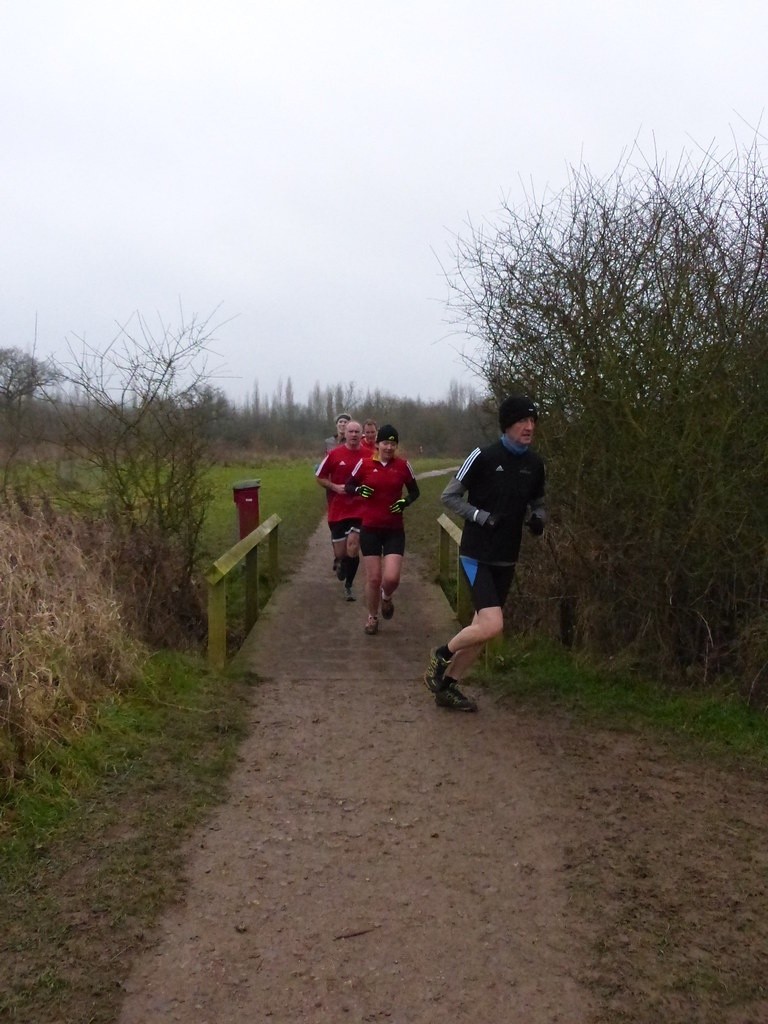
[422,647,452,693]
[336,562,344,580]
[365,618,378,634]
[435,684,477,711]
[344,589,356,602]
[380,592,394,620]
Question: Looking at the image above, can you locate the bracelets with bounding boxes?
[473,510,479,521]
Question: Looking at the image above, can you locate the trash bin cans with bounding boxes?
[231,478,261,540]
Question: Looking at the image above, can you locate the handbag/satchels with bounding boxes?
[527,513,543,535]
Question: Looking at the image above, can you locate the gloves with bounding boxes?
[486,511,508,529]
[390,499,407,513]
[355,485,374,498]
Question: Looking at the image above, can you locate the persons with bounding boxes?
[344,424,421,634]
[423,397,547,714]
[315,422,374,602]
[316,414,351,572]
[361,420,379,451]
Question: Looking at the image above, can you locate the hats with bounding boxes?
[500,396,539,432]
[376,425,399,444]
